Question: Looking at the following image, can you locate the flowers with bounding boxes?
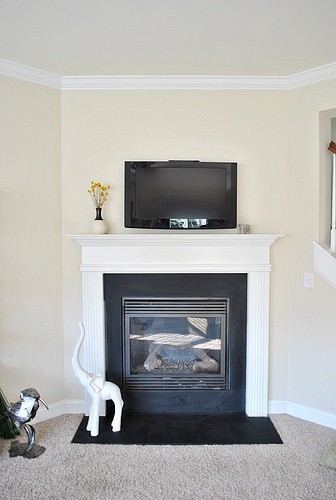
[87,180,111,208]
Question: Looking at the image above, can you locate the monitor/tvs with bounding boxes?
[124,160,237,230]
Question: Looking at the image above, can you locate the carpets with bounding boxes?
[71,415,284,445]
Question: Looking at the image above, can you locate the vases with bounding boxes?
[91,209,107,234]
